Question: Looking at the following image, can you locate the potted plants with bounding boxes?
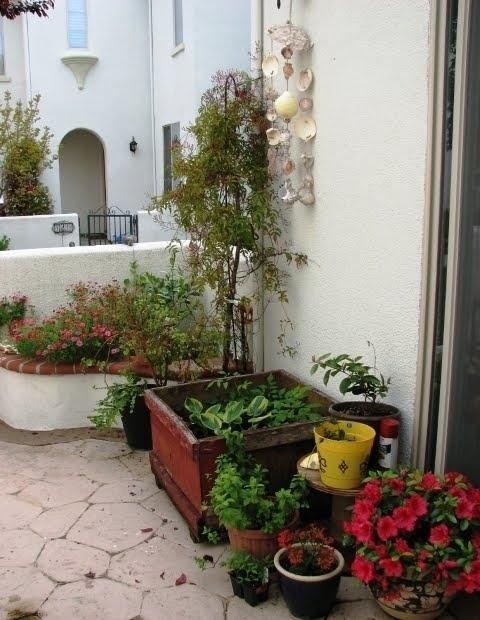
[89,339,402,620]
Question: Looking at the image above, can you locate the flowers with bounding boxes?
[344,465,480,601]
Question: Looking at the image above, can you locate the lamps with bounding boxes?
[130,136,137,154]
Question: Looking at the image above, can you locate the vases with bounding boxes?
[365,569,460,619]
